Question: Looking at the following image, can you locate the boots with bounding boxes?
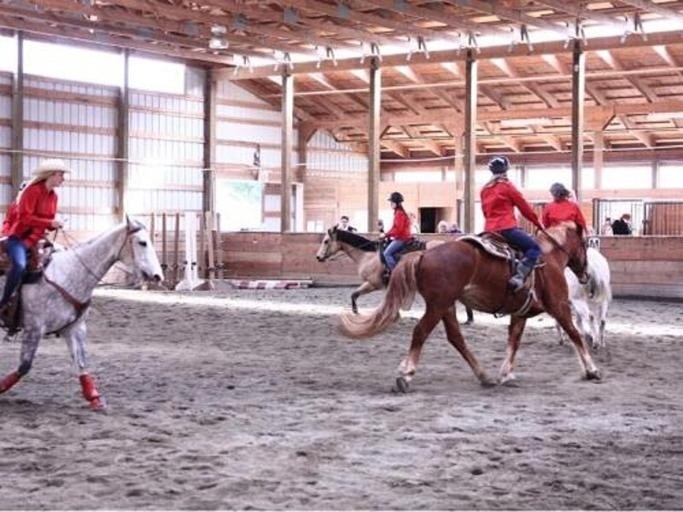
[508,257,531,290]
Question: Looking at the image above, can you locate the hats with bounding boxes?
[33,160,69,172]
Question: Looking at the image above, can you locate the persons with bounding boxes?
[612,215,632,235]
[0,158,73,331]
[480,156,564,289]
[333,215,355,231]
[437,220,448,233]
[601,214,614,235]
[450,222,460,233]
[541,181,589,247]
[375,219,385,231]
[376,191,412,282]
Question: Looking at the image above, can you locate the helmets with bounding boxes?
[387,192,403,202]
[488,157,508,173]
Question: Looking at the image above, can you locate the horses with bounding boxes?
[316,226,473,325]
[0,212,164,411]
[337,221,602,393]
[556,247,611,353]
